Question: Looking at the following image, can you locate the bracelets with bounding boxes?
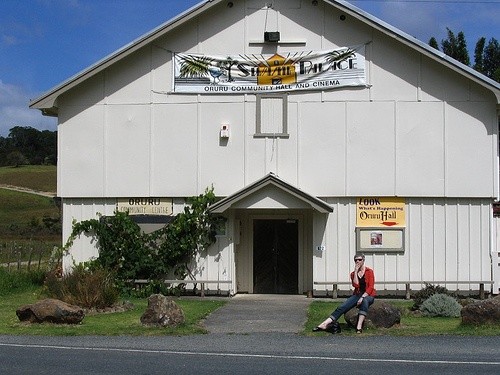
[361,296,364,299]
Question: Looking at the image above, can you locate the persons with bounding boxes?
[312,253,377,334]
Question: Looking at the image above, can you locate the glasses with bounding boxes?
[354,258,363,263]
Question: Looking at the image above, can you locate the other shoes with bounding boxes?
[312,326,328,331]
[356,328,362,334]
